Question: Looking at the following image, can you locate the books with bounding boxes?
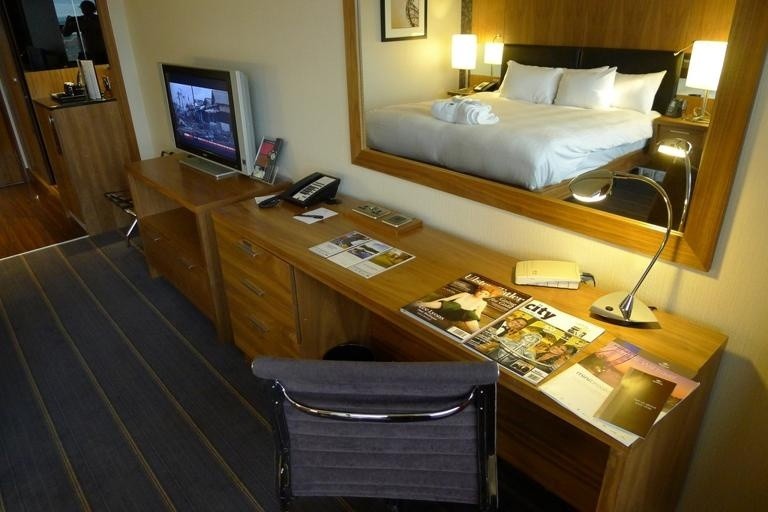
[462,300,606,385]
[540,338,701,446]
[400,272,532,343]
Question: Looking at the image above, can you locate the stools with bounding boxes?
[104,190,137,248]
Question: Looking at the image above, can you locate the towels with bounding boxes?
[431,96,499,125]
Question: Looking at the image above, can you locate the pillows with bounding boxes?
[497,60,668,115]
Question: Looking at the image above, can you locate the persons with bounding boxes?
[493,316,572,366]
[413,285,501,333]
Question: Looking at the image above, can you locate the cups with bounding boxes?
[73,83,86,95]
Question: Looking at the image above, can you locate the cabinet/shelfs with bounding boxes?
[123,164,232,344]
[33,99,133,237]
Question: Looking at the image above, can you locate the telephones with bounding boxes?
[473,81,498,92]
[279,172,341,208]
[665,97,685,118]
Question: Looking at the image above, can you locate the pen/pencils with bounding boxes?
[294,214,324,219]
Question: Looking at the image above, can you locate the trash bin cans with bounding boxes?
[323,344,376,362]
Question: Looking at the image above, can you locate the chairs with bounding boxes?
[252,353,497,512]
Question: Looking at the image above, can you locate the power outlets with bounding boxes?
[352,204,391,219]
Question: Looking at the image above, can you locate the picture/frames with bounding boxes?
[380,0,427,43]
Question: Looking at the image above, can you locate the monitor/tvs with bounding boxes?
[156,59,256,180]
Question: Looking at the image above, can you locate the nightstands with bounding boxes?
[649,114,708,217]
[448,87,474,96]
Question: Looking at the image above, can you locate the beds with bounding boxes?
[363,43,684,200]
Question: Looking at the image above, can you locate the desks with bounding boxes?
[211,188,729,511]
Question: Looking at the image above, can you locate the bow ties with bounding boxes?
[501,326,507,333]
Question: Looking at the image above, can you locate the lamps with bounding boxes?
[656,137,693,232]
[568,169,673,329]
[484,42,504,77]
[683,41,727,123]
[452,34,477,93]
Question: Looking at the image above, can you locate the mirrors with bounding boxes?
[677,51,716,99]
[470,42,503,78]
[344,1,764,273]
